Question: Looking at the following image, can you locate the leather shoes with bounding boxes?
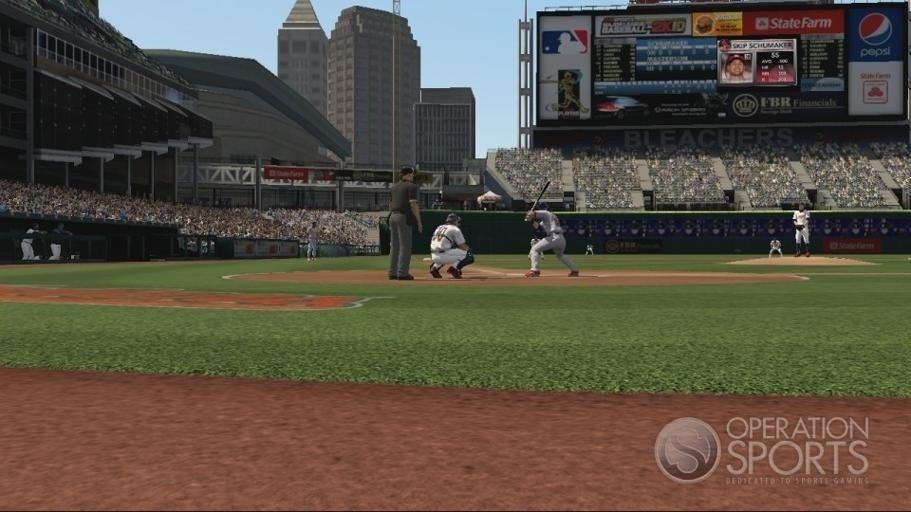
[397,273,414,280]
[389,275,396,280]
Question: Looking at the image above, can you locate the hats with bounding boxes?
[446,212,462,223]
[400,167,413,176]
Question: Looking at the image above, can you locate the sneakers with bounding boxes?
[567,269,579,277]
[446,266,462,279]
[525,269,540,278]
[430,266,442,279]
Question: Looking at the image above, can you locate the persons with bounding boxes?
[558,33,586,54]
[552,72,590,113]
[721,54,751,83]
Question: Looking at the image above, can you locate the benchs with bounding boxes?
[489,143,911,212]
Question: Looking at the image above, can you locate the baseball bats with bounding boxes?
[524,182,550,221]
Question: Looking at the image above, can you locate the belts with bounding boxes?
[554,232,558,234]
[439,250,444,253]
